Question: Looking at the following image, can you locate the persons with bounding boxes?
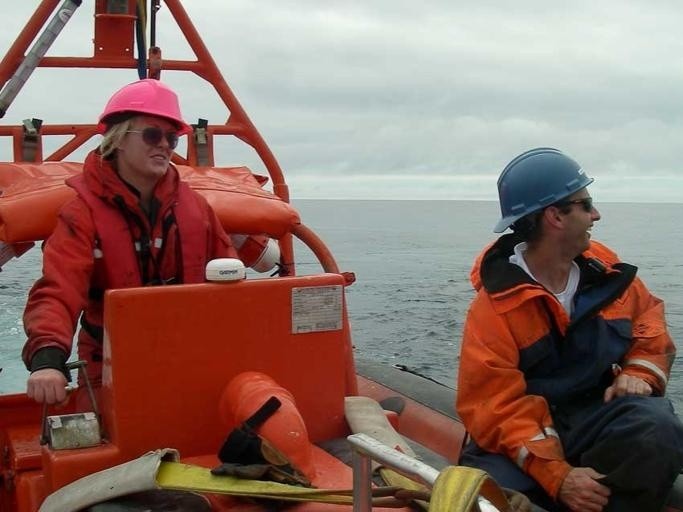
[19,80,241,408]
[452,145,682,512]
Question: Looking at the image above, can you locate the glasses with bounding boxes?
[557,196,594,213]
[121,128,179,150]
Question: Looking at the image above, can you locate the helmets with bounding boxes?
[96,77,193,136]
[492,146,595,235]
